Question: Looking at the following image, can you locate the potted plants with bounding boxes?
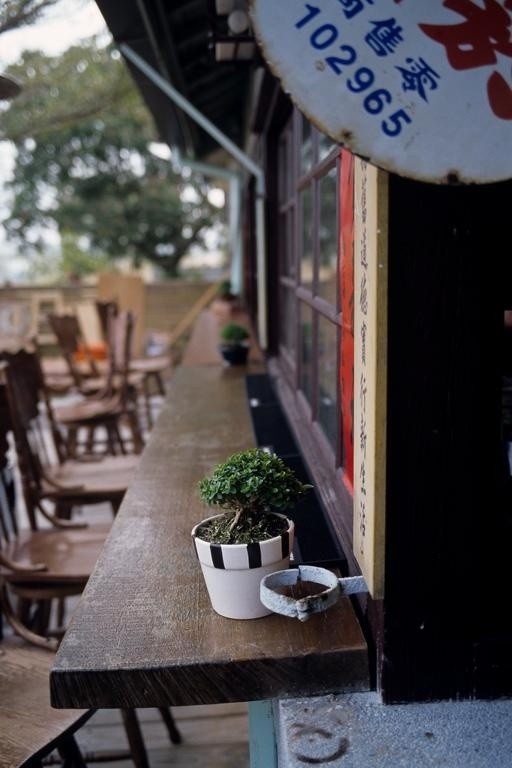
[219,325,251,363]
[190,448,314,626]
